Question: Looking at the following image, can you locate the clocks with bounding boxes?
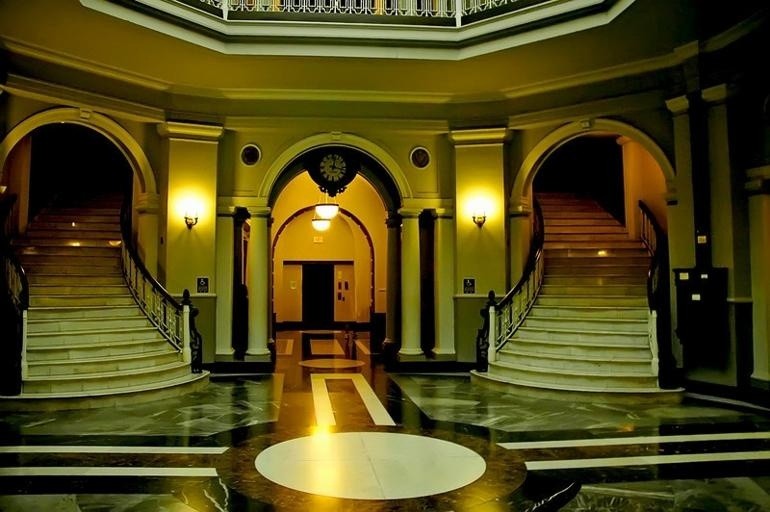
[305,147,361,198]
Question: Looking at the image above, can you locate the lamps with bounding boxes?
[311,212,331,232]
[314,188,340,221]
[183,216,198,230]
[472,215,487,228]
